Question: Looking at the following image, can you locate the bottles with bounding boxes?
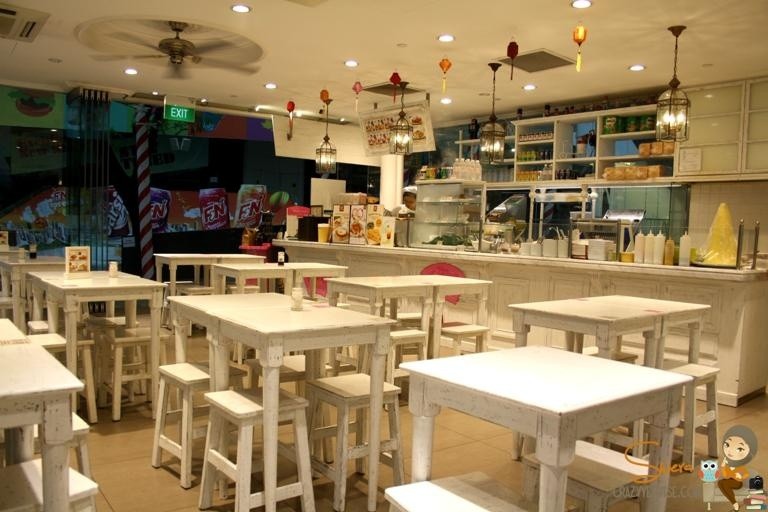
[678,231,689,267]
[646,229,655,264]
[150,187,172,234]
[555,164,576,180]
[29,230,37,260]
[635,229,644,263]
[665,234,674,265]
[199,188,230,231]
[517,150,552,161]
[518,132,555,142]
[419,159,484,181]
[233,184,266,228]
[655,228,664,265]
[277,249,285,266]
[516,164,552,180]
[101,186,130,236]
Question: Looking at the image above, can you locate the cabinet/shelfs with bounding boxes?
[511,104,678,189]
[272,238,768,409]
[411,181,488,252]
[671,75,768,183]
[453,130,515,189]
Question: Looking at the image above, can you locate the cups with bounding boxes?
[17,247,25,259]
[290,287,305,312]
[28,243,38,259]
[317,223,330,244]
[277,251,285,267]
[107,260,118,278]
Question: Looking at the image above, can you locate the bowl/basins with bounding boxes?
[471,239,491,252]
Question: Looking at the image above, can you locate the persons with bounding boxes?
[399,186,417,214]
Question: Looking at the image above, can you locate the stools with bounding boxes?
[176,285,212,337]
[440,322,490,356]
[198,387,317,512]
[331,345,368,377]
[307,371,404,512]
[26,333,98,423]
[152,360,247,490]
[522,439,651,511]
[0,458,100,512]
[103,324,175,421]
[384,473,526,512]
[385,313,422,332]
[0,296,27,334]
[83,316,145,403]
[246,354,334,477]
[385,326,429,411]
[664,362,719,471]
[583,344,648,458]
[26,319,65,332]
[230,285,260,295]
[161,281,194,326]
[31,412,90,480]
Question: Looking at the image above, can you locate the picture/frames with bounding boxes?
[65,246,95,278]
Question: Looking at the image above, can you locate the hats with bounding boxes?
[402,185,417,195]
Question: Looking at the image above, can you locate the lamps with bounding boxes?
[390,81,413,156]
[316,98,337,174]
[656,25,692,141]
[480,62,505,165]
